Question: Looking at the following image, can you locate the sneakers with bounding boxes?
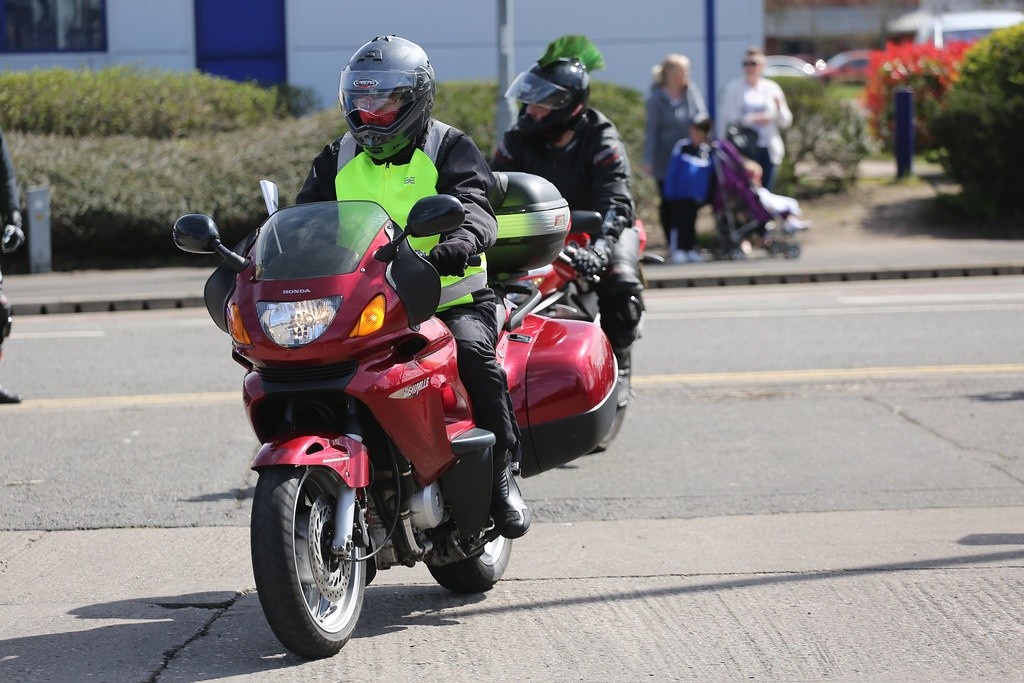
[487,464,531,540]
[672,250,688,262]
[615,352,632,407]
[688,249,706,263]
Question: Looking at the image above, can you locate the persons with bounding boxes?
[642,51,710,265]
[490,56,644,412]
[744,157,813,236]
[294,35,532,539]
[659,111,712,265]
[259,179,279,223]
[0,126,24,405]
[713,46,793,259]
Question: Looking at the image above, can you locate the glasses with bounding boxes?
[351,98,399,109]
[741,61,761,66]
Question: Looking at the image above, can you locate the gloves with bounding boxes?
[571,244,610,280]
[430,239,475,277]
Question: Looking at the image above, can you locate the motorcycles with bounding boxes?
[169,194,519,658]
[525,209,644,450]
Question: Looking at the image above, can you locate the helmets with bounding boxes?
[504,58,592,141]
[338,37,434,158]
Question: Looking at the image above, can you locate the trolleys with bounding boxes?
[707,136,808,261]
